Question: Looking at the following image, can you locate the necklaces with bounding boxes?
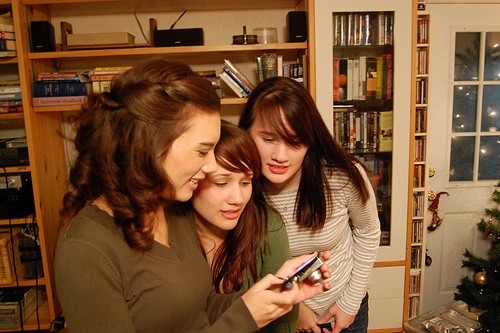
[270,188,297,211]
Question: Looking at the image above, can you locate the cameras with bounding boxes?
[282,252,324,290]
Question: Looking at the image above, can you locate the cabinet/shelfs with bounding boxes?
[0,0,430,333]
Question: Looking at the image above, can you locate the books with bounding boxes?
[0,18,28,147]
[218,59,255,98]
[257,52,307,88]
[408,20,429,319]
[193,71,221,98]
[333,12,394,153]
[32,66,133,107]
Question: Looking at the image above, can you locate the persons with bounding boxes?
[190,119,301,333]
[54,59,332,333]
[239,77,381,333]
